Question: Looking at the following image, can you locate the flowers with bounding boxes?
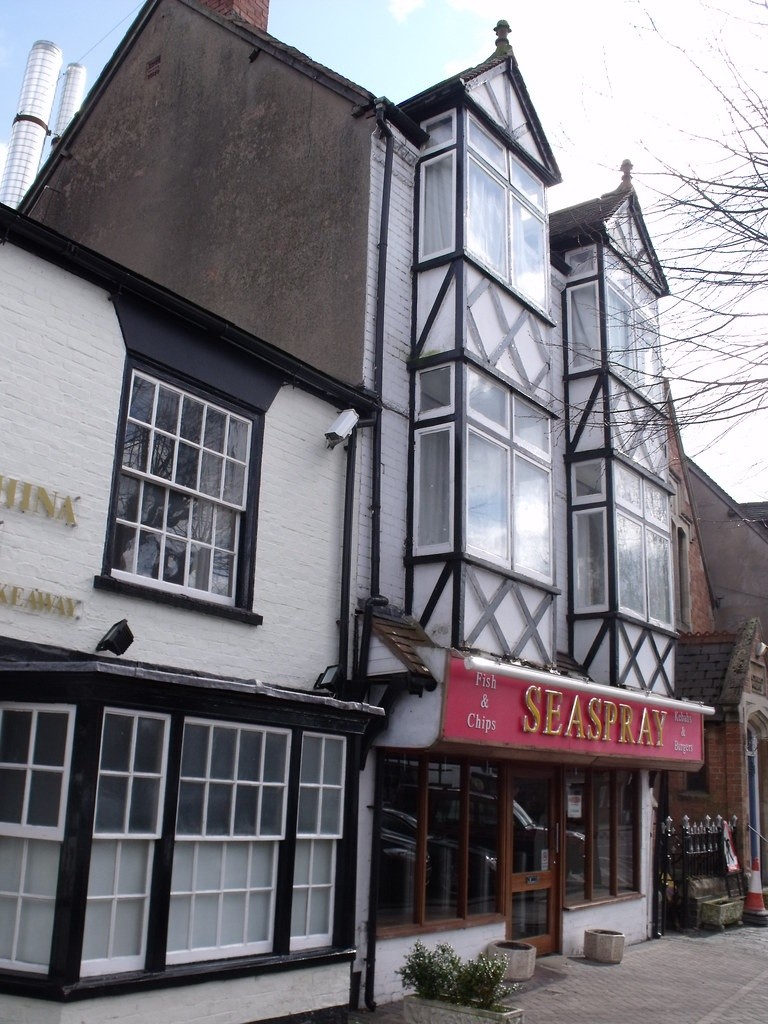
[392,936,523,1011]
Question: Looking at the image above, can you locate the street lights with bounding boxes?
[742,856,768,926]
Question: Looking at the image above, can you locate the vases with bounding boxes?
[487,940,537,983]
[584,928,626,964]
[702,898,744,930]
[403,992,524,1024]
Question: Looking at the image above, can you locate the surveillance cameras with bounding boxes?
[324,408,360,444]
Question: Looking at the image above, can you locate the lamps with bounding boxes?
[313,664,346,693]
[95,618,134,656]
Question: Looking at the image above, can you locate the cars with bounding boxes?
[376,785,585,923]
